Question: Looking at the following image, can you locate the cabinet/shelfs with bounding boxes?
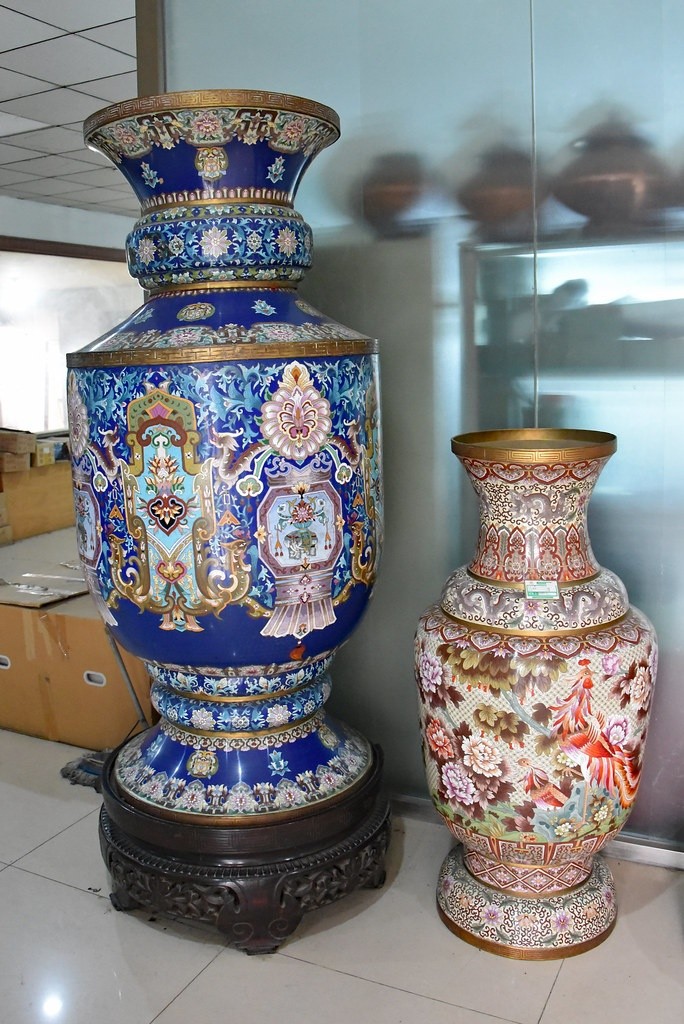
[456,230,683,845]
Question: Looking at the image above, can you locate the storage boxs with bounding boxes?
[0,427,157,762]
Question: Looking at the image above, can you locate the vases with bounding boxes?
[65,90,394,827]
[415,429,661,965]
